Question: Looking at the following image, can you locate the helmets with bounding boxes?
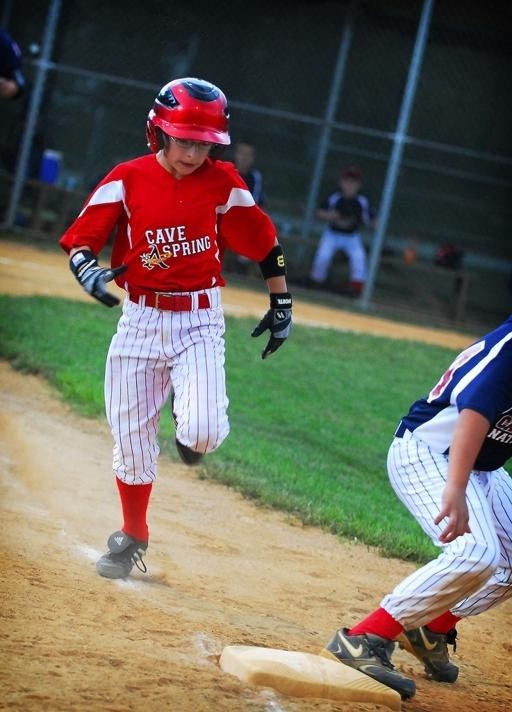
[145,75,231,155]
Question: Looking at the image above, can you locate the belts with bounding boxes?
[128,287,210,312]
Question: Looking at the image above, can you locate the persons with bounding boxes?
[0,28,29,178]
[57,76,292,580]
[323,316,511,698]
[311,166,371,297]
[224,142,267,275]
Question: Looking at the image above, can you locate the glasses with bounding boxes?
[170,133,210,152]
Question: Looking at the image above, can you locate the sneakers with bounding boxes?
[170,392,206,461]
[325,625,416,701]
[399,625,459,683]
[96,529,147,579]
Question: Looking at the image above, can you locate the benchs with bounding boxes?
[0,174,478,324]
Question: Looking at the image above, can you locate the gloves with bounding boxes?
[69,248,126,305]
[253,292,293,358]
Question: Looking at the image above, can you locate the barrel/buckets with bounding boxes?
[37,148,63,182]
